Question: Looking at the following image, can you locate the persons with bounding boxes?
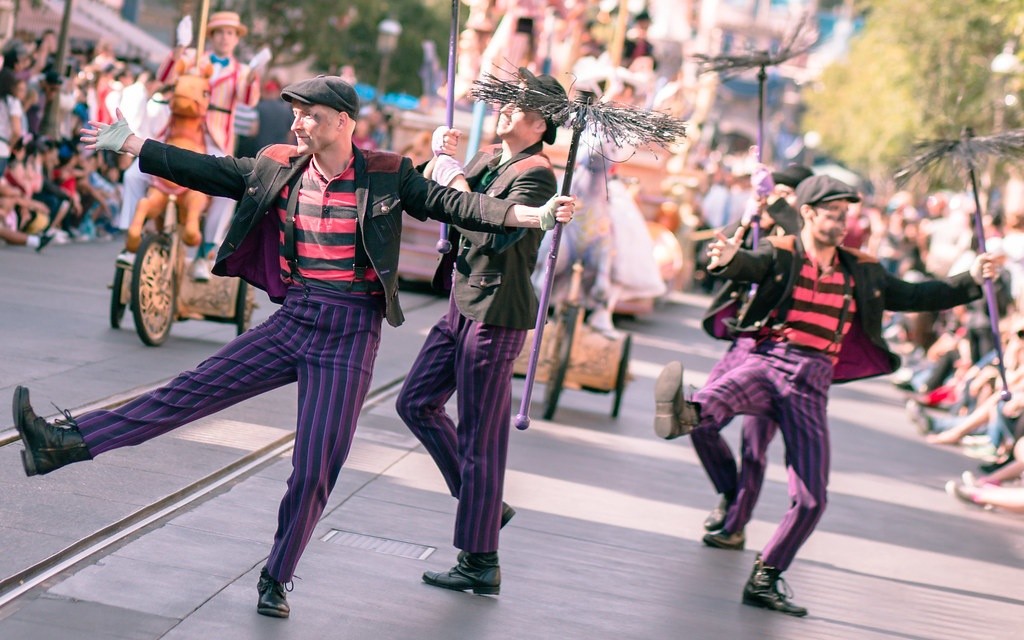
[656,175,1004,616]
[115,11,272,281]
[239,81,292,154]
[11,75,575,619]
[0,30,175,252]
[699,173,742,290]
[356,108,392,150]
[844,180,1024,511]
[395,68,570,595]
[689,166,814,550]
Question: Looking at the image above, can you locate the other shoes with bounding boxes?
[905,399,933,434]
[35,234,54,253]
[945,481,996,513]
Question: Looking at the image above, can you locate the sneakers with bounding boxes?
[118,251,137,264]
[191,258,210,282]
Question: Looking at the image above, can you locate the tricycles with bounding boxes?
[514,249,636,420]
[109,170,259,348]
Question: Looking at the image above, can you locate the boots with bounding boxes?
[421,549,502,596]
[742,552,808,617]
[11,386,94,478]
[653,361,703,441]
[703,497,732,532]
[257,567,291,619]
[501,500,515,533]
[702,529,746,551]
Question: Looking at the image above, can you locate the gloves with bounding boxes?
[248,48,270,73]
[176,15,194,49]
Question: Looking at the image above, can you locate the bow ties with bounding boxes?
[210,55,229,66]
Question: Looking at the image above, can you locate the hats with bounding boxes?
[794,174,863,206]
[517,66,570,146]
[772,163,815,194]
[206,11,248,38]
[280,74,360,122]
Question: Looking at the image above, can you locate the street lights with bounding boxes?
[376,21,404,109]
[981,41,1020,195]
